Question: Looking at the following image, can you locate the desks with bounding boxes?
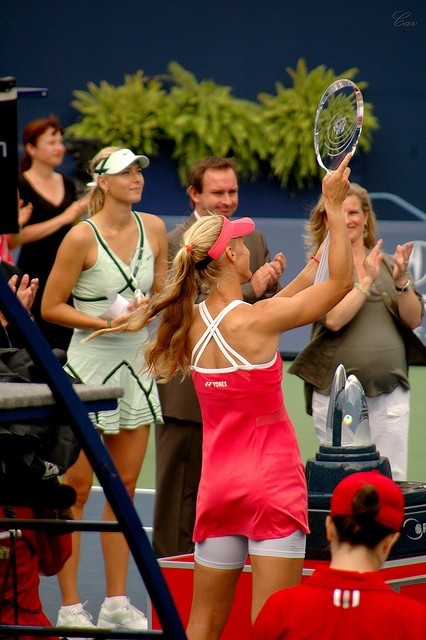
[0,383,126,422]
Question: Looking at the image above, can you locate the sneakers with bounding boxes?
[98,602,149,632]
[56,599,99,640]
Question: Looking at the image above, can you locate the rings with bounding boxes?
[362,261,368,269]
[403,259,410,266]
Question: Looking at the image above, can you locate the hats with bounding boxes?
[330,470,405,532]
[191,214,255,271]
[86,147,150,187]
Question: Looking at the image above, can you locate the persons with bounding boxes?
[245,470,426,640]
[124,151,362,640]
[7,113,94,351]
[0,191,35,267]
[286,181,425,481]
[2,273,38,331]
[0,505,72,640]
[41,145,169,640]
[80,152,353,640]
[151,154,286,558]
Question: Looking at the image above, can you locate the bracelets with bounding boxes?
[106,320,113,332]
[354,283,372,299]
[395,279,413,295]
[311,254,321,265]
